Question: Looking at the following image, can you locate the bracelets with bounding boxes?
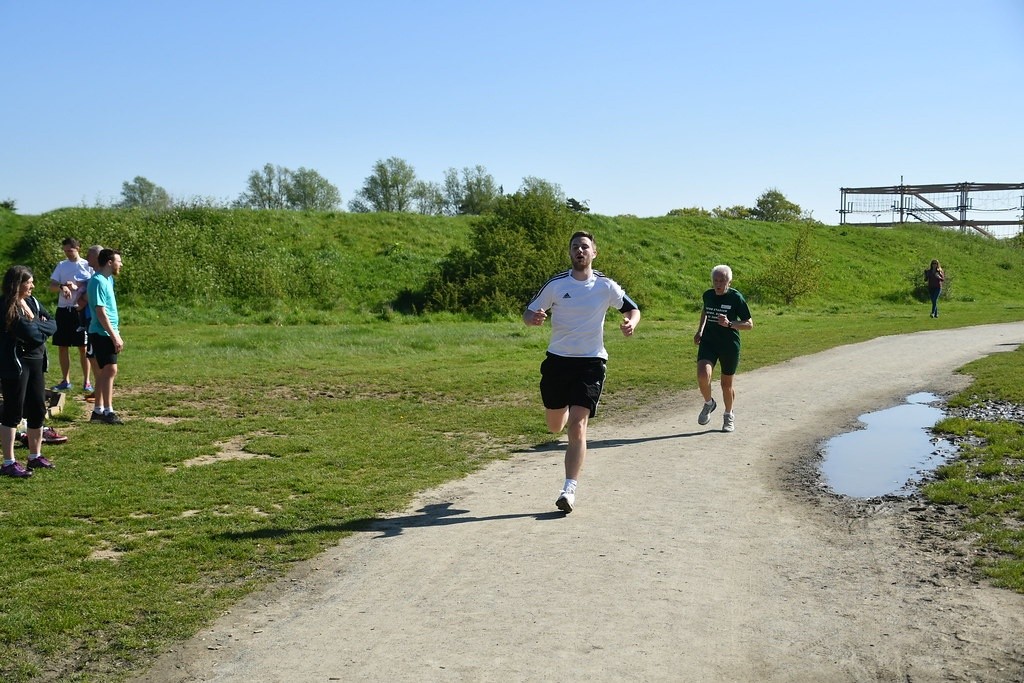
[60,284,67,288]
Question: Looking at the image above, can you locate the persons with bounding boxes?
[66,271,91,331]
[925,260,944,318]
[77,245,106,402]
[50,238,94,392]
[87,249,124,423]
[0,266,53,478]
[524,231,641,514]
[693,265,753,432]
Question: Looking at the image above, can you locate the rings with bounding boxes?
[536,322,538,324]
[537,319,539,320]
[629,332,631,334]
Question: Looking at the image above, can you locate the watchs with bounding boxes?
[728,322,733,327]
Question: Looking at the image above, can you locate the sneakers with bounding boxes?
[52,380,71,390]
[722,412,735,433]
[102,411,124,426]
[19,432,45,447]
[84,384,94,392]
[27,456,55,471]
[42,427,68,443]
[698,398,716,425]
[91,410,104,423]
[556,489,575,513]
[1,461,32,477]
